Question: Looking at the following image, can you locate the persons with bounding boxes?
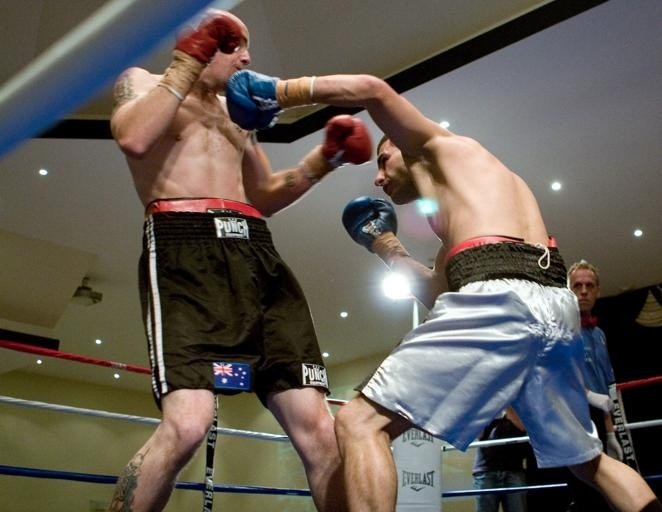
[113,10,372,512]
[564,259,624,511]
[473,406,527,512]
[226,68,662,512]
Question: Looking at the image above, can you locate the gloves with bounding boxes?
[227,69,281,130]
[175,12,241,63]
[323,115,371,167]
[341,196,398,253]
